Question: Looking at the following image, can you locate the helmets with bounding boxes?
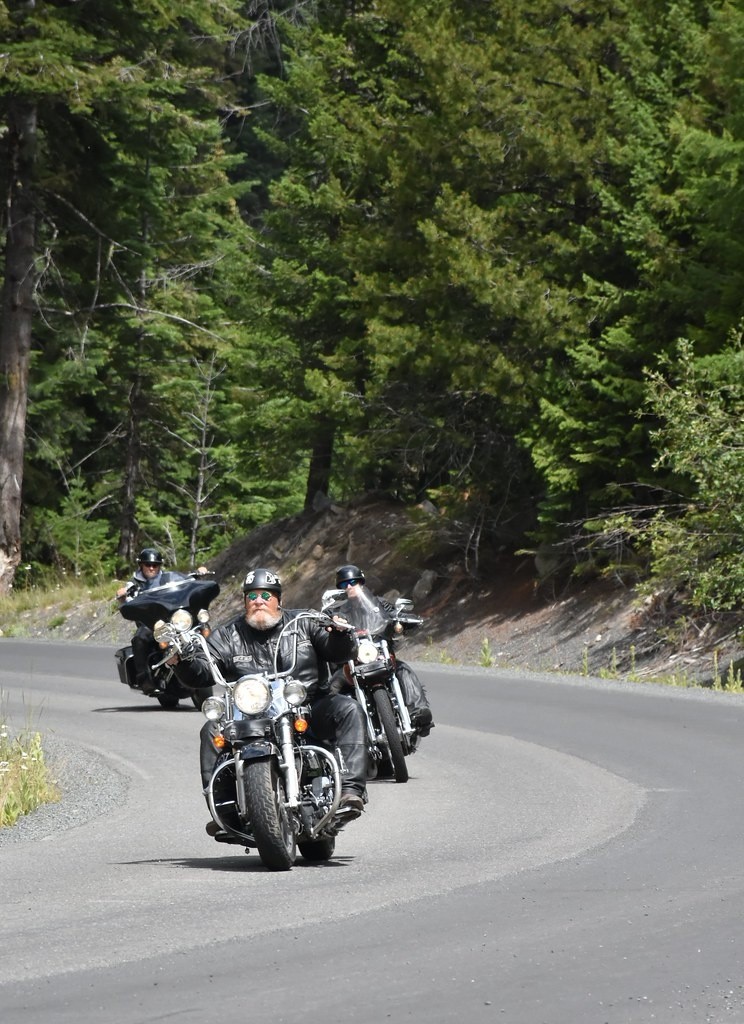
[335,565,366,589]
[137,548,164,564]
[242,568,282,593]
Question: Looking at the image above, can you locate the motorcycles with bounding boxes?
[112,570,216,711]
[149,619,365,867]
[314,597,437,784]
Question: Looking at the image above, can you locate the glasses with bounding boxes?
[142,563,159,568]
[247,591,272,600]
[338,580,359,589]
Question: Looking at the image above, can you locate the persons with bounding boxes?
[116,548,208,695]
[162,569,368,836]
[322,565,432,738]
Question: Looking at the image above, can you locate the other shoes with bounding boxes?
[205,820,225,837]
[415,707,433,738]
[339,793,365,812]
